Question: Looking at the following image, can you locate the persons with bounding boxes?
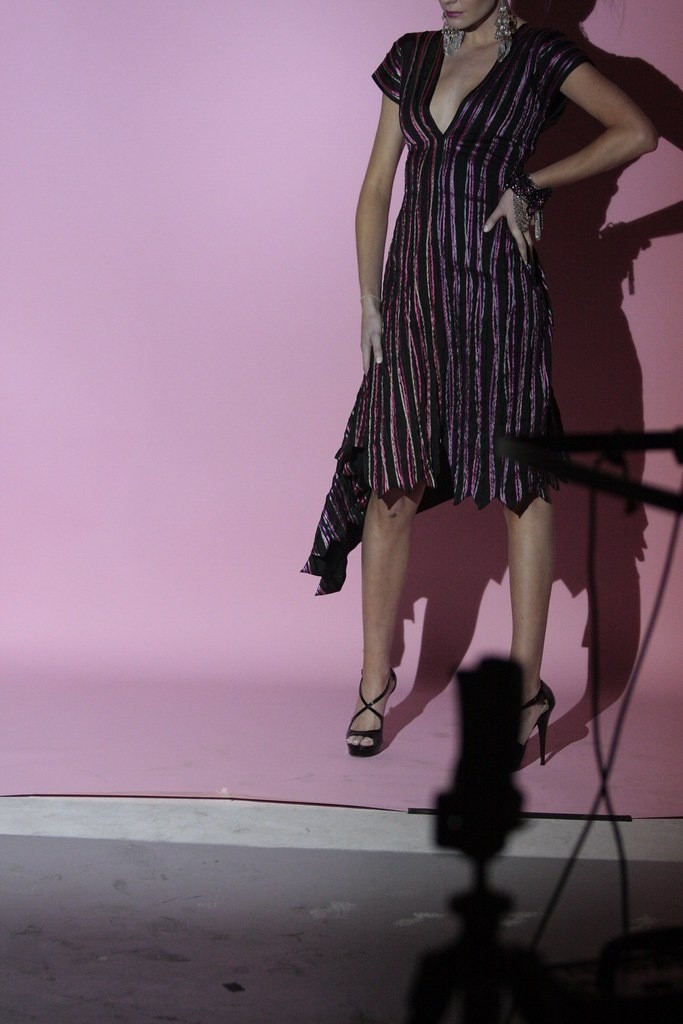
[300,0,659,774]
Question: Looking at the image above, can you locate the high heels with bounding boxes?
[505,679,555,775]
[345,665,397,756]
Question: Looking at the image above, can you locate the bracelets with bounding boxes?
[361,295,382,302]
[508,177,552,241]
